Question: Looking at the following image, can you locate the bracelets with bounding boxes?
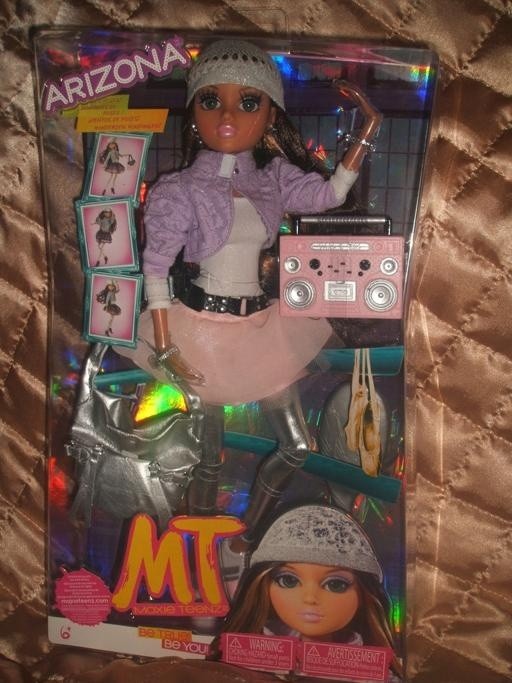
[157,346,178,363]
[351,137,376,153]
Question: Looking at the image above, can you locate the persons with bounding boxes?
[82,132,132,353]
[215,504,398,680]
